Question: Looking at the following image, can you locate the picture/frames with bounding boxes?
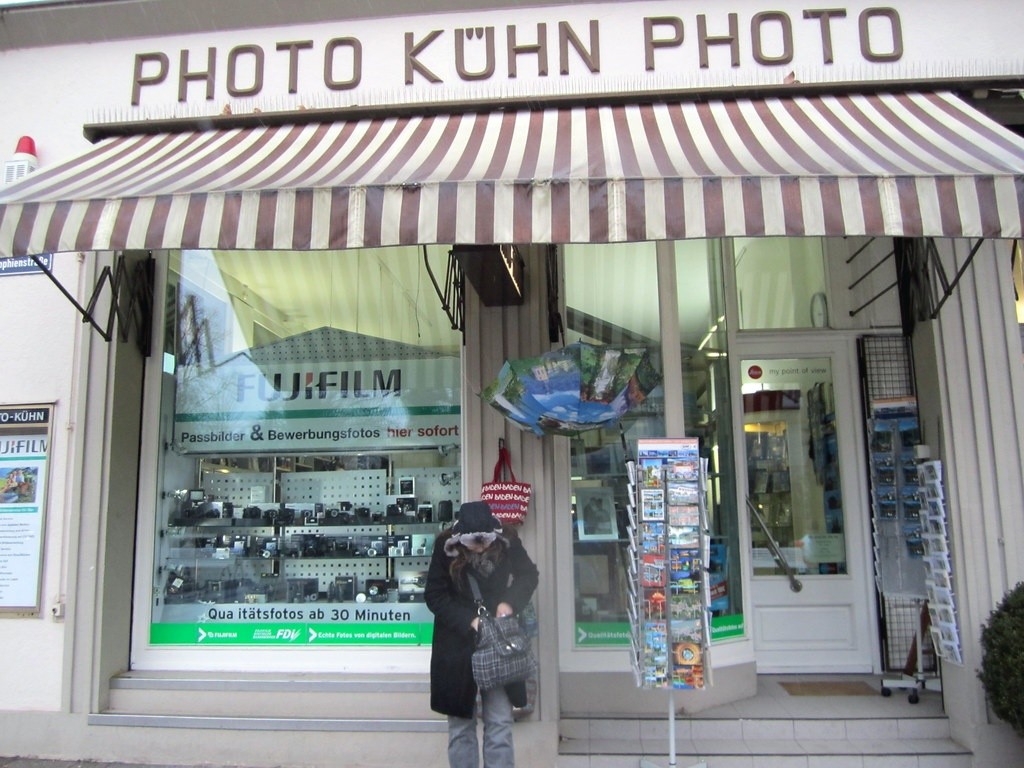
[571,438,585,476]
[575,486,618,540]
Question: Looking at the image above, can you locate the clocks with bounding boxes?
[810,292,828,328]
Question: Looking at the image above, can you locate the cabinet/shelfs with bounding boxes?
[392,466,460,580]
[748,467,793,530]
[279,470,386,594]
[166,472,276,583]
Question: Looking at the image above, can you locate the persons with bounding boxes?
[423,502,540,768]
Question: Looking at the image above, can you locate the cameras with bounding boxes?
[195,533,435,559]
[168,490,435,524]
[167,565,426,603]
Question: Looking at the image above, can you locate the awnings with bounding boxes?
[0,92,1024,255]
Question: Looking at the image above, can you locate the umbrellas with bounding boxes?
[475,312,664,438]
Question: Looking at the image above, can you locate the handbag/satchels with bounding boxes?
[470,602,538,692]
[481,447,531,526]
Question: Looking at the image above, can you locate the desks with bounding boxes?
[574,539,631,622]
